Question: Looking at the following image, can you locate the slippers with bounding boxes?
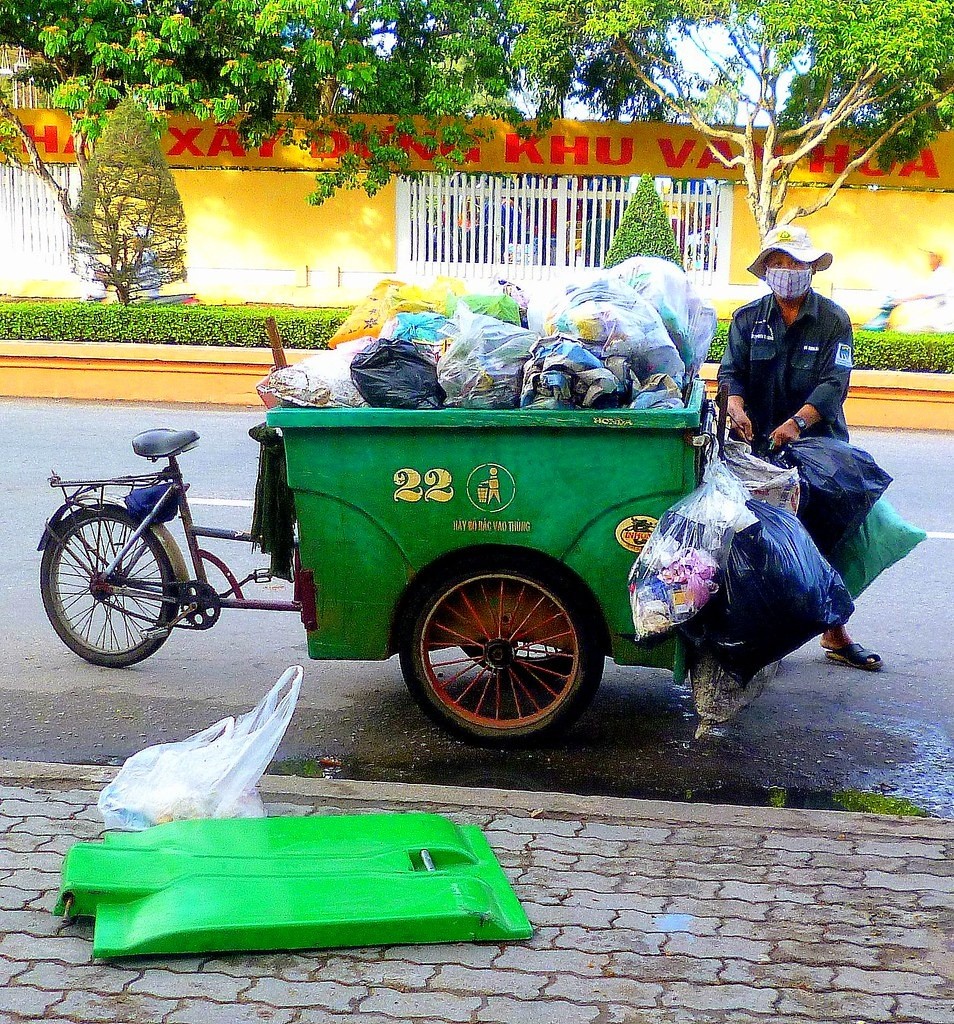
[825,643,884,669]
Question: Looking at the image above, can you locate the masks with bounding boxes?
[762,264,813,299]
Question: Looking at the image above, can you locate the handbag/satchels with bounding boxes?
[99,664,303,834]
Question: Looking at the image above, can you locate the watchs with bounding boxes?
[791,415,807,430]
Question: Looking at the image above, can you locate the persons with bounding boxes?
[894,244,954,333]
[116,227,159,304]
[715,225,885,671]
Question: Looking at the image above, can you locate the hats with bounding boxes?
[745,226,833,281]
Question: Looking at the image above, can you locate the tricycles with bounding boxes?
[34,375,716,748]
